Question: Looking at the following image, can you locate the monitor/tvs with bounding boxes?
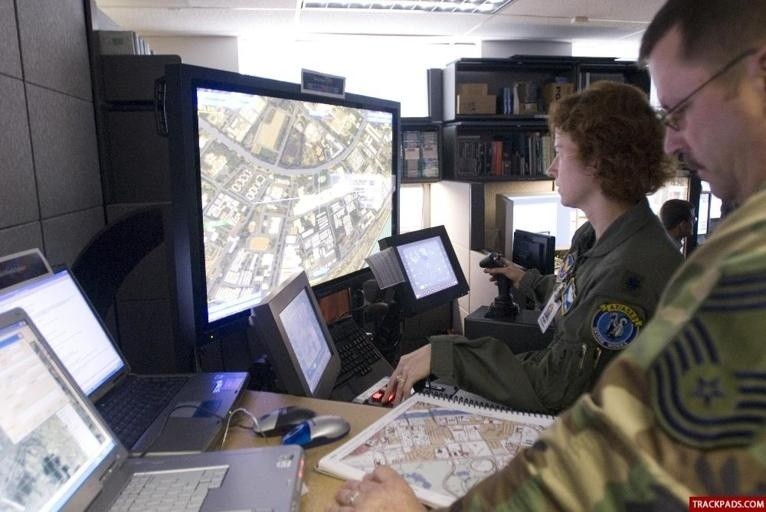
[252,269,343,401]
[165,60,402,350]
[511,227,556,312]
[375,224,470,320]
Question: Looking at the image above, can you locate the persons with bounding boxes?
[660,199,695,249]
[381,80,686,414]
[338,0,766,512]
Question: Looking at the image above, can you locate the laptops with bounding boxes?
[0,307,305,511]
[0,262,250,451]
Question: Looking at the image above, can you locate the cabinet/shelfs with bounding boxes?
[448,56,653,179]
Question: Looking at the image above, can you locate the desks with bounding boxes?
[208,391,392,512]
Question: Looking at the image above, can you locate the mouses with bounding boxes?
[251,405,316,438]
[281,413,351,451]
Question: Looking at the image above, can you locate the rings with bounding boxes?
[350,491,361,505]
[396,374,406,384]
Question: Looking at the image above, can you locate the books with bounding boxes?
[457,140,504,176]
[514,132,555,177]
[498,81,538,115]
[316,390,560,511]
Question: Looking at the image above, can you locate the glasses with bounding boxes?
[656,49,756,132]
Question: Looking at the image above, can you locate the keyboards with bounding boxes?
[327,318,394,403]
